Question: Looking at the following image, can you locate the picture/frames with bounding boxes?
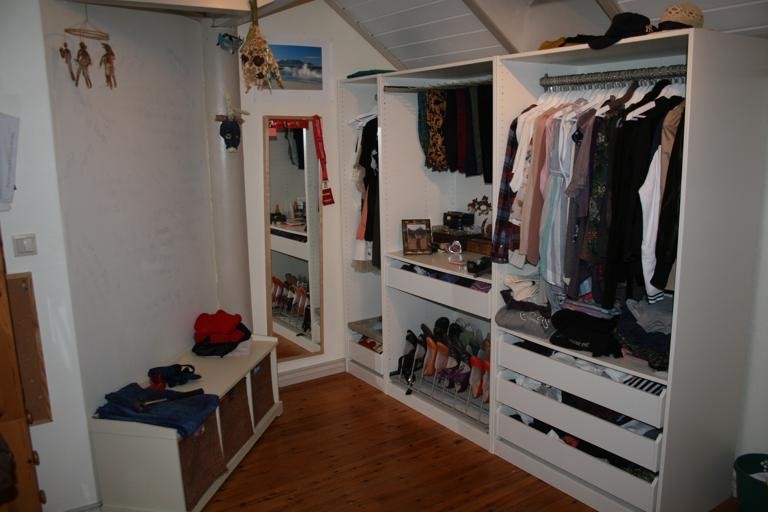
[254,36,332,99]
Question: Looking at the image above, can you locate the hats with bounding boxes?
[605,12,651,38]
[660,2,704,28]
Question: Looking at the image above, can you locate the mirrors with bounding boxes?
[262,115,325,364]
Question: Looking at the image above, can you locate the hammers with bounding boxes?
[133,388,203,412]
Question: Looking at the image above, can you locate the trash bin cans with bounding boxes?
[734,453,768,512]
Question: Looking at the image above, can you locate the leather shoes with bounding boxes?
[272,274,310,331]
[401,317,490,403]
